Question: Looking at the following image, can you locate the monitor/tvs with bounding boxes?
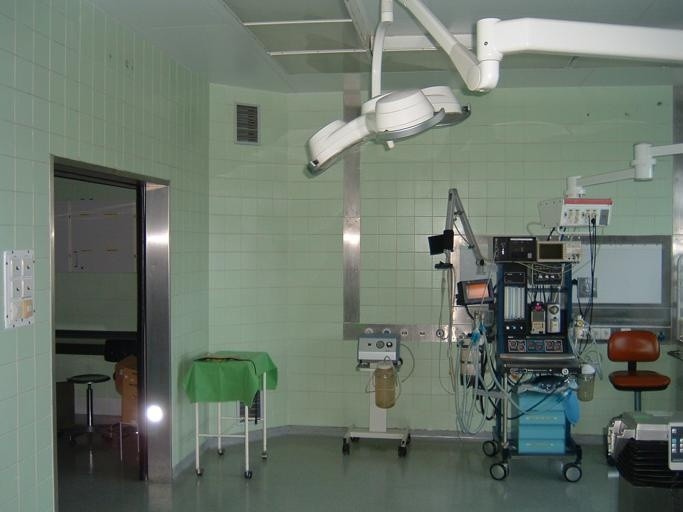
[668,422,683,471]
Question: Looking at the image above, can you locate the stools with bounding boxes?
[65,372,113,449]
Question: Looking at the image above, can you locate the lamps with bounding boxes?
[306,0,682,177]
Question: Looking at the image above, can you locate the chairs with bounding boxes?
[604,330,670,411]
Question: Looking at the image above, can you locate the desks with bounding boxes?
[189,350,272,480]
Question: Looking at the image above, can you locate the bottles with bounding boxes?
[577,365,595,402]
[374,361,396,409]
